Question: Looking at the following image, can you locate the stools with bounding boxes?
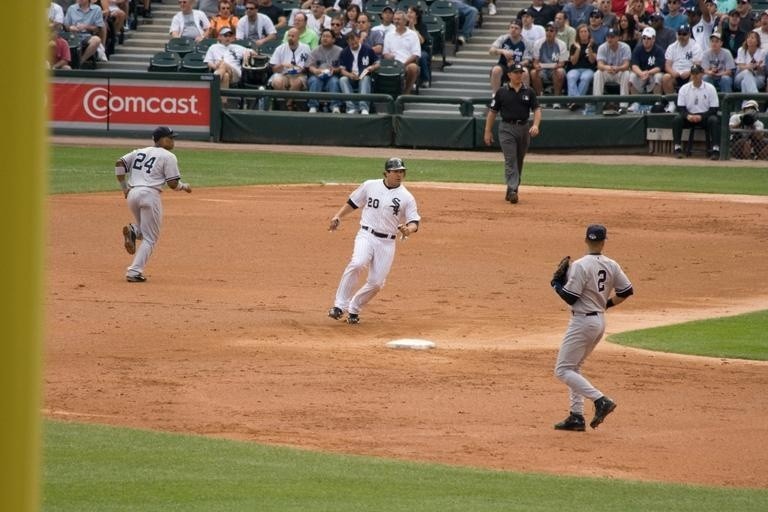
[684,123,712,159]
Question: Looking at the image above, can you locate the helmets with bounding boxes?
[385,157,408,172]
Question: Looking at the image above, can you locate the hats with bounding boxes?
[585,225,609,240]
[510,1,768,40]
[151,126,182,142]
[690,64,704,73]
[506,64,525,74]
[219,28,234,35]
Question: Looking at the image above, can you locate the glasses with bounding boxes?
[331,23,342,27]
[246,7,256,10]
[220,7,231,10]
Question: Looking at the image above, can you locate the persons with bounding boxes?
[113,123,192,282]
[170,0,497,115]
[548,223,633,433]
[44,1,129,70]
[495,0,767,161]
[321,155,421,324]
[482,61,544,204]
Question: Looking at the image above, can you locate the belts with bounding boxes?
[361,224,399,240]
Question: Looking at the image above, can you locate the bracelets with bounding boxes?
[119,180,127,190]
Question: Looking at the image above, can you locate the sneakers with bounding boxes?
[327,306,343,321]
[122,223,136,256]
[555,412,586,432]
[125,273,147,282]
[538,100,677,114]
[590,396,617,430]
[347,312,360,324]
[508,189,519,204]
[710,148,722,161]
[286,101,370,113]
[675,146,684,159]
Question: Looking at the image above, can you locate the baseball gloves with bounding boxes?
[551,255,571,286]
